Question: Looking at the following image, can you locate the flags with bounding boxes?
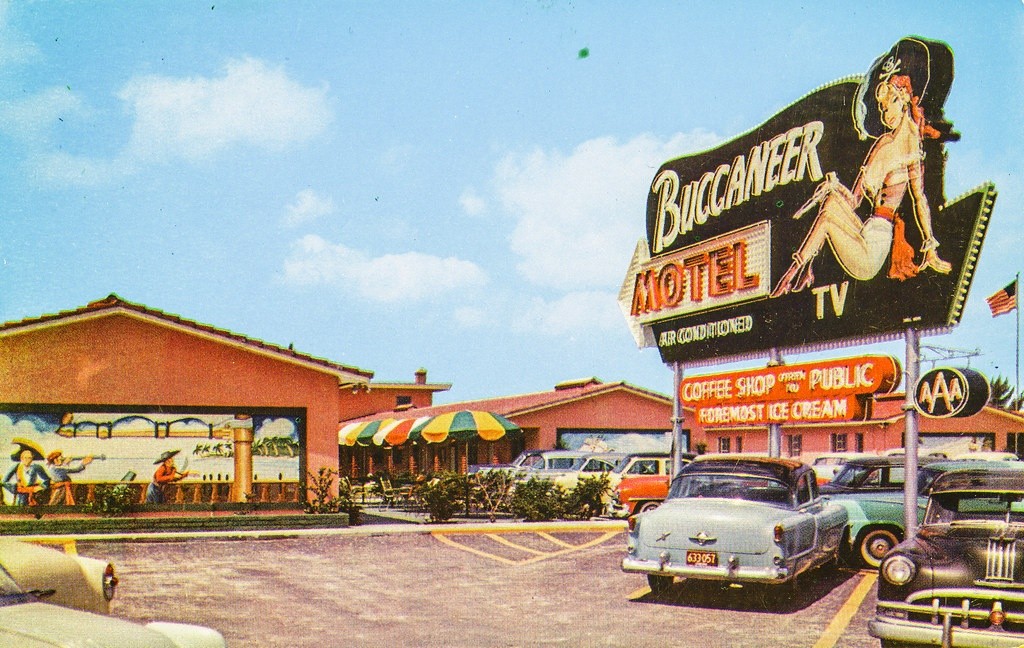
[986,276,1016,316]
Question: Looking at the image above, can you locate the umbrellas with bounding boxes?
[422,409,520,476]
[336,418,440,475]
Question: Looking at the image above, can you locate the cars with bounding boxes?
[556,453,629,513]
[809,451,1020,570]
[868,470,1024,648]
[620,452,849,600]
[603,453,700,518]
[467,449,590,487]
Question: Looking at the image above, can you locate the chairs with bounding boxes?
[378,476,408,511]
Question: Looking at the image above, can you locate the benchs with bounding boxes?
[695,484,790,504]
